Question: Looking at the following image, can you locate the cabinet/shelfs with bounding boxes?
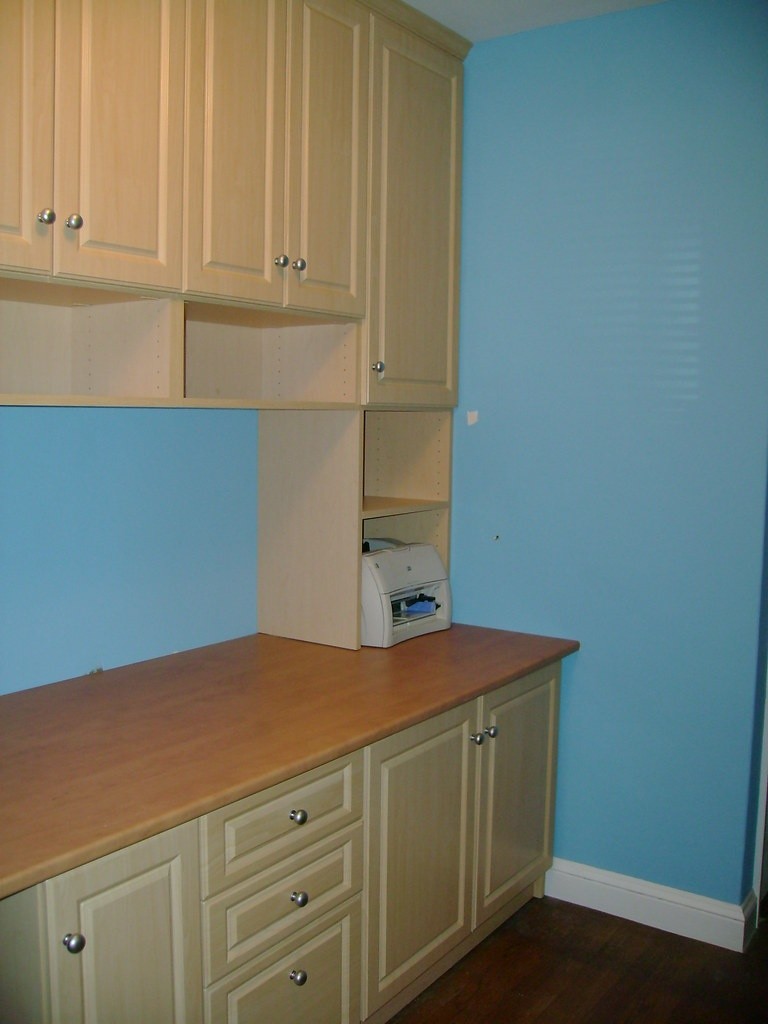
[1,0,186,299]
[0,622,579,1024]
[263,0,474,412]
[186,0,370,330]
